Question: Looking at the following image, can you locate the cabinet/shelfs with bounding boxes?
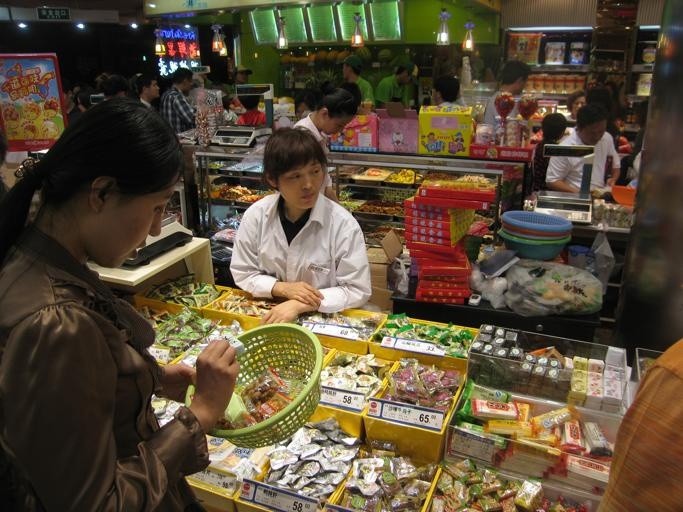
[195,146,528,272]
[502,25,660,152]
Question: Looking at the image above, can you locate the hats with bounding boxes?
[401,62,416,80]
[232,65,253,77]
[337,55,362,70]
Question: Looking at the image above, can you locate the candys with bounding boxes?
[518,93,538,145]
[494,91,514,146]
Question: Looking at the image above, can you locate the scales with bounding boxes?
[210,84,272,146]
[124,214,192,265]
[533,144,595,224]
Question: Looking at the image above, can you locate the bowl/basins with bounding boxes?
[496,221,572,261]
[610,184,636,206]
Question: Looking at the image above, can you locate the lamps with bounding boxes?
[351,19,364,48]
[437,17,450,46]
[212,28,224,52]
[154,31,166,54]
[462,28,474,52]
[219,41,228,56]
[277,24,289,49]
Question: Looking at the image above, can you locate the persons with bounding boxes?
[61,48,460,202]
[230,124,373,325]
[485,62,649,202]
[1,97,242,512]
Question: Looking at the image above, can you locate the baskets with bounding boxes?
[497,211,572,260]
[185,323,323,447]
